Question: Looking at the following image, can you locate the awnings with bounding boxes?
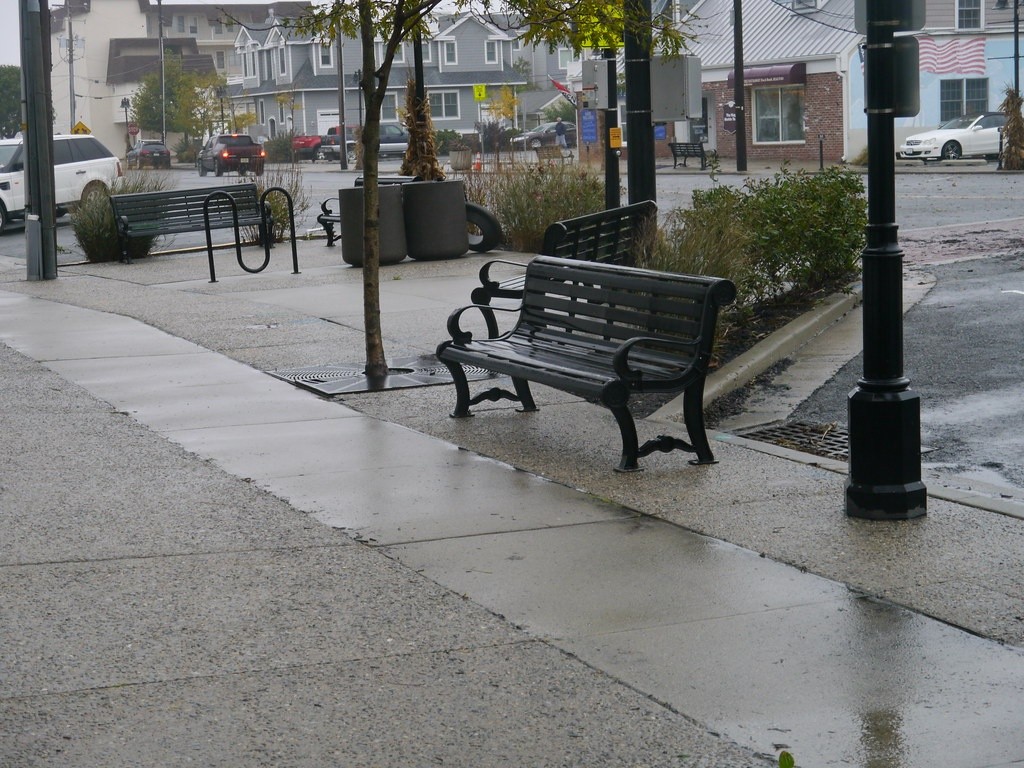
[727,63,807,89]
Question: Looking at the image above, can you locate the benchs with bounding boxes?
[475,200,660,340]
[667,142,717,170]
[438,255,737,471]
[110,182,273,265]
[533,145,575,166]
[317,175,422,247]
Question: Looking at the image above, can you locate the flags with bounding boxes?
[551,79,577,105]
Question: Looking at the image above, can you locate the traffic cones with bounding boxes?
[473,152,481,171]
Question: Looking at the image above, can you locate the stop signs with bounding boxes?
[128,124,139,135]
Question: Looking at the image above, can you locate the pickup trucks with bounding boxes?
[197,133,265,178]
[292,125,359,160]
[321,122,409,163]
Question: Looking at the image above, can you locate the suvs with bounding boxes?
[126,140,171,171]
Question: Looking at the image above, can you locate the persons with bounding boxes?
[556,117,567,149]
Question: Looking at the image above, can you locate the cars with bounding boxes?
[510,122,577,150]
[899,111,1010,160]
[0,135,126,234]
